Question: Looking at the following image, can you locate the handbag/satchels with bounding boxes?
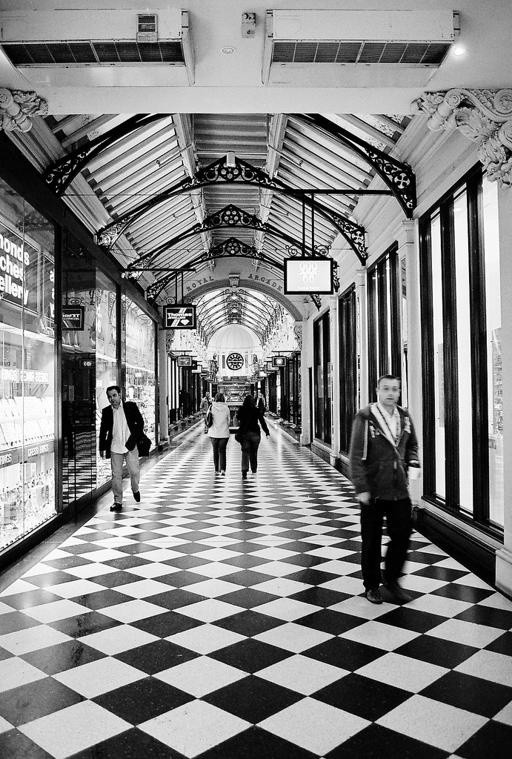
[204,412,214,427]
[235,426,250,443]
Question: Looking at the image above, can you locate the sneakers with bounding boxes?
[214,468,259,480]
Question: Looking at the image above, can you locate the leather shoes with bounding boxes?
[358,578,414,604]
[109,490,141,511]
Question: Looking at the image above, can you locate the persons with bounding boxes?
[347,374,424,604]
[207,393,231,475]
[100,386,151,511]
[199,392,212,434]
[256,394,266,408]
[235,395,271,479]
